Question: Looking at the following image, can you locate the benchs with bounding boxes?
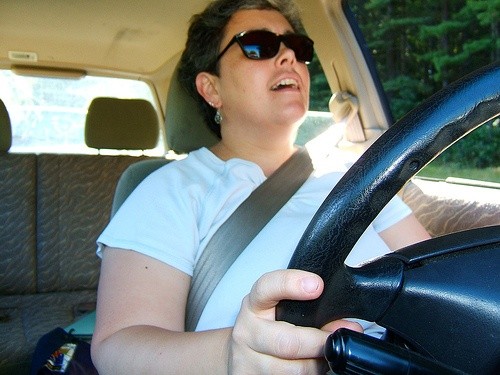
[0,96,179,368]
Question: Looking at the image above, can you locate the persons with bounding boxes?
[91,0,431,375]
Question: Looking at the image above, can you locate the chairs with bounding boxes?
[113,54,218,226]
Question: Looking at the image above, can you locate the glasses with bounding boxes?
[211,28,314,64]
